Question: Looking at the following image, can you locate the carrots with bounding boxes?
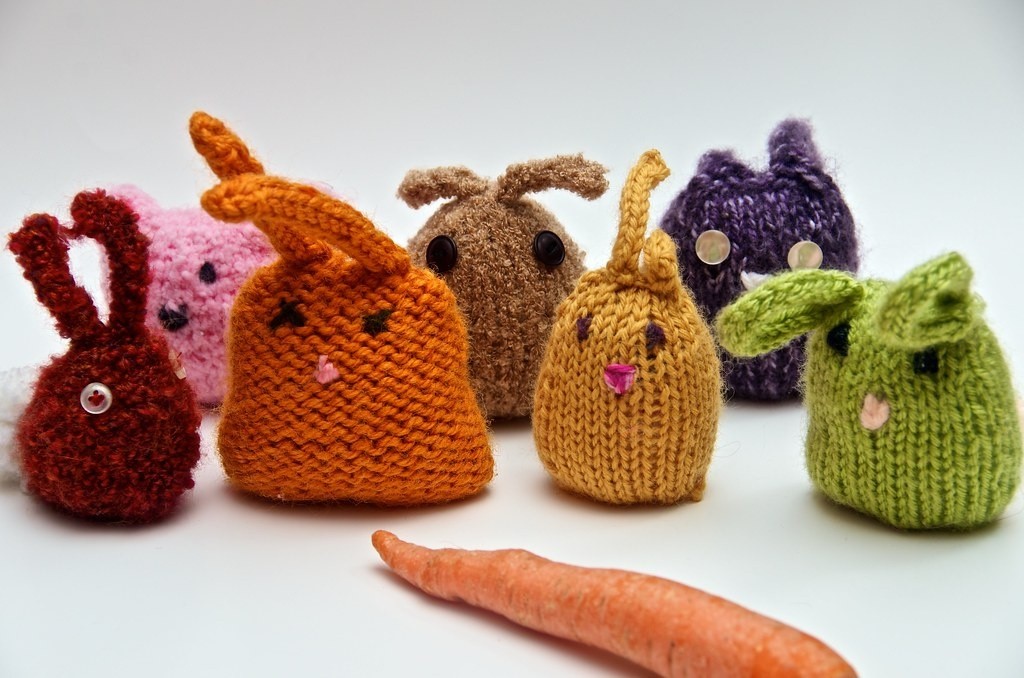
[372,530,858,678]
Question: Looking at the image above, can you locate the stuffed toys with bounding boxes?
[396,156,608,415]
[96,182,281,406]
[189,110,496,506]
[5,187,203,526]
[658,117,861,405]
[714,252,1024,532]
[533,148,723,505]
